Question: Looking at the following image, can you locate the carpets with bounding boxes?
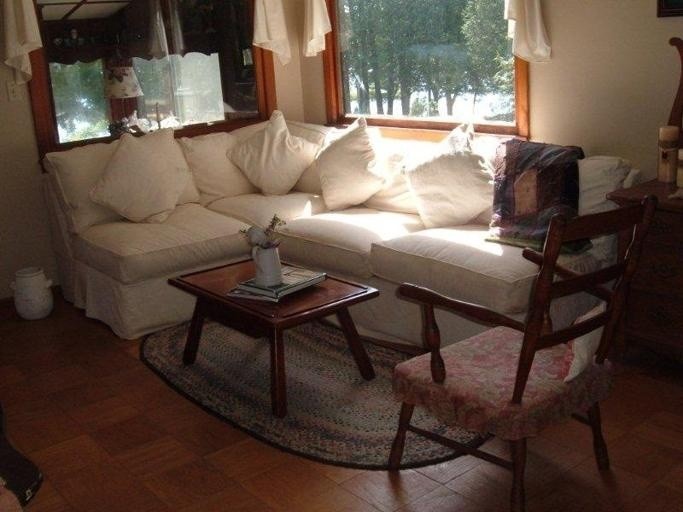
[139,320,497,471]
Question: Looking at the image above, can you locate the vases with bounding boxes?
[250,247,283,285]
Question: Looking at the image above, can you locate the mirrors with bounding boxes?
[24,0,278,175]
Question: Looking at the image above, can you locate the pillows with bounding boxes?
[402,122,494,229]
[88,133,201,223]
[44,128,199,235]
[362,138,504,223]
[576,156,630,217]
[483,138,594,256]
[225,108,322,196]
[286,121,347,195]
[314,116,407,214]
[180,120,272,208]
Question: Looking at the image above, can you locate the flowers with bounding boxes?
[238,210,287,249]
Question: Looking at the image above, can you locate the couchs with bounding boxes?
[43,119,643,352]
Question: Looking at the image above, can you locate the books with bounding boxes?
[225,287,281,304]
[239,265,328,299]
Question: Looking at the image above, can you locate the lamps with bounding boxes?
[104,65,144,118]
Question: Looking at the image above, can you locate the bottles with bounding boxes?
[676,149,683,188]
[657,126,679,184]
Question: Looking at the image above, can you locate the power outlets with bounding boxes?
[6,80,22,103]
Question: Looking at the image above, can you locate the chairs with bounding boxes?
[387,193,659,511]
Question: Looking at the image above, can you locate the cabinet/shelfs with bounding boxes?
[604,178,682,367]
